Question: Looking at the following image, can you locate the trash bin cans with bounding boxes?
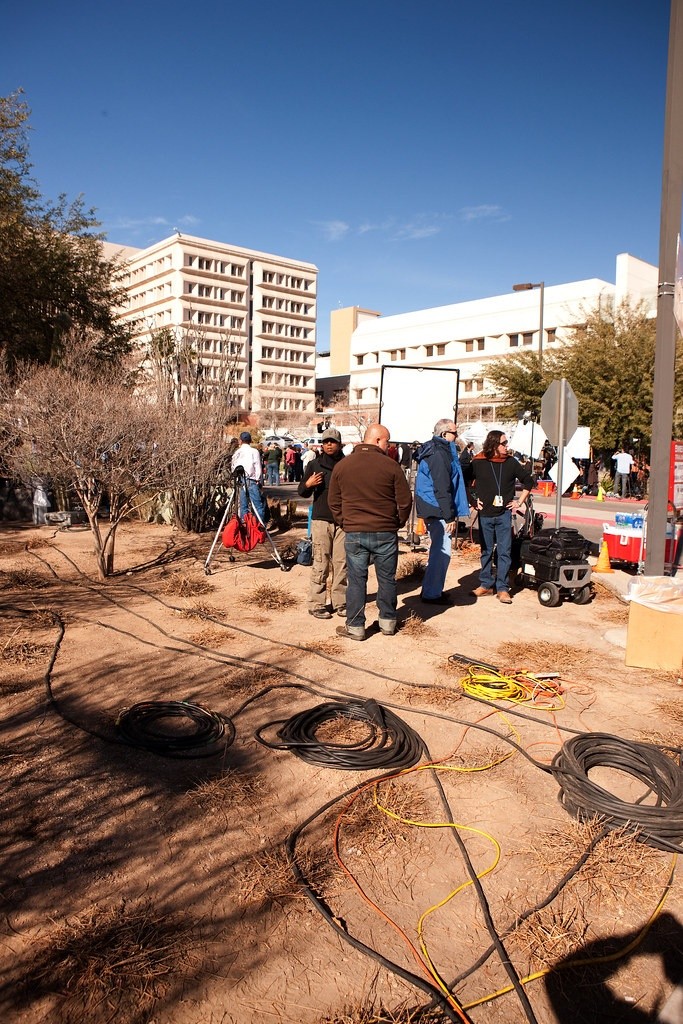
[34,504,47,525]
[625,577,682,673]
[537,480,554,497]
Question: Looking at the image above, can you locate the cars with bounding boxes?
[259,435,296,450]
[301,437,323,453]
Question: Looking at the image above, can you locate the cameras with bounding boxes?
[232,466,244,477]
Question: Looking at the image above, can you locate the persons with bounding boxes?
[514,452,532,484]
[228,434,279,534]
[258,442,282,486]
[297,429,347,618]
[283,442,324,482]
[413,419,470,604]
[613,448,635,498]
[328,424,414,641]
[393,441,420,483]
[465,430,533,602]
[343,440,353,456]
[460,442,485,508]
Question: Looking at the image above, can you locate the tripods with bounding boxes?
[204,475,288,571]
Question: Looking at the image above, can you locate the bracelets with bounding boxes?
[517,501,522,507]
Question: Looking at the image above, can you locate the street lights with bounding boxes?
[511,281,545,376]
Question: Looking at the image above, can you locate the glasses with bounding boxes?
[499,440,508,446]
[440,430,458,436]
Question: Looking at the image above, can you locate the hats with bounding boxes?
[239,432,251,442]
[321,428,341,443]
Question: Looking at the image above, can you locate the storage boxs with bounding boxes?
[601,522,680,564]
[624,576,683,673]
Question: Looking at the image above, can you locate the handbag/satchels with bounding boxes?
[295,539,313,566]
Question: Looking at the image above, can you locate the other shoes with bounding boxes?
[336,626,365,641]
[420,591,454,606]
[308,608,331,619]
[374,619,394,635]
[337,607,347,617]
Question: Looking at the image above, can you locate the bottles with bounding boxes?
[616,512,643,528]
[666,500,674,535]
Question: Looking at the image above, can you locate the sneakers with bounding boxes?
[469,585,493,597]
[496,589,511,603]
